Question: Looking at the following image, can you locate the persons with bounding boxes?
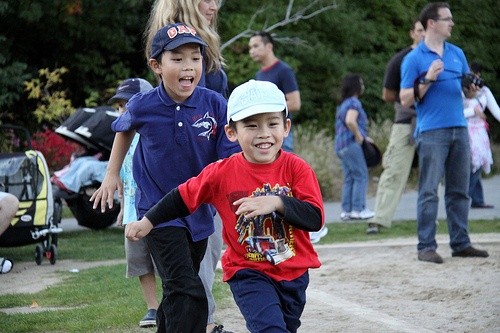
[125,78,327,333]
[107,78,163,326]
[0,191,20,273]
[139,0,227,333]
[458,59,499,210]
[333,73,375,221]
[89,23,245,333]
[367,19,428,234]
[243,30,328,246]
[398,0,491,262]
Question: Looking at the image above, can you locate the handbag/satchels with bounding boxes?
[361,139,382,167]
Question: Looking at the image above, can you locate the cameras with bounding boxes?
[460,71,484,94]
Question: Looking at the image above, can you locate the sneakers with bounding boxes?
[139,309,159,327]
[341,210,376,223]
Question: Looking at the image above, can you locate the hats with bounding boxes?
[226,78,289,125]
[151,23,208,58]
[109,78,152,103]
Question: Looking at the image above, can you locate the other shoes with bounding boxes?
[471,203,494,209]
[419,247,490,262]
[367,223,379,232]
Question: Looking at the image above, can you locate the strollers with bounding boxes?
[0,124,65,264]
